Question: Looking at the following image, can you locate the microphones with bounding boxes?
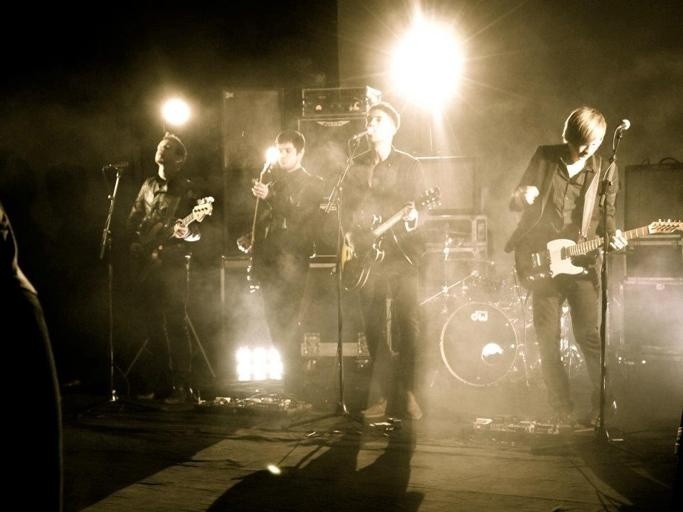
[617,119,631,139]
[353,127,374,140]
[102,159,128,171]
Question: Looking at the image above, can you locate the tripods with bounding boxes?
[76,232,168,418]
[119,249,218,389]
[286,201,392,433]
[531,199,652,464]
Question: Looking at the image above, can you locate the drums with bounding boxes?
[439,300,520,389]
[446,260,497,294]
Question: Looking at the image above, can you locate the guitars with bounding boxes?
[137,196,216,260]
[341,187,442,292]
[515,218,682,290]
[246,147,280,292]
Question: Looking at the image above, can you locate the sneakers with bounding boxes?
[360,399,392,417]
[400,390,422,419]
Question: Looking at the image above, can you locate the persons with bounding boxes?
[0,208,63,512]
[340,101,433,423]
[124,132,201,405]
[235,128,322,399]
[503,106,628,436]
